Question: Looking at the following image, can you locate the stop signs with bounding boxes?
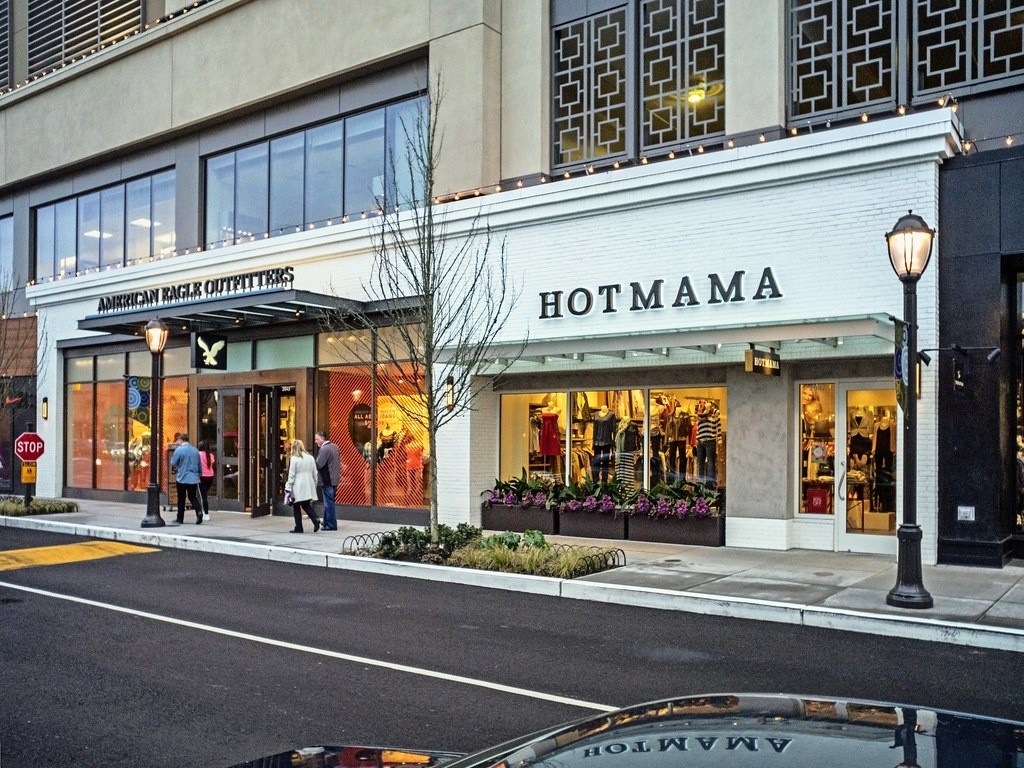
[15,432,45,462]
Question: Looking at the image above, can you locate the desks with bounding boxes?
[804,480,867,531]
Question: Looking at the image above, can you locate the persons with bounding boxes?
[802,384,823,413]
[171,434,215,524]
[593,405,640,500]
[663,403,722,492]
[848,405,874,468]
[286,440,321,534]
[362,423,424,505]
[871,417,896,495]
[540,402,562,456]
[315,431,340,531]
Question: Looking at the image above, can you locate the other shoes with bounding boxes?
[289,526,303,534]
[196,511,203,524]
[322,521,336,531]
[314,518,320,532]
[172,518,183,525]
[203,514,210,521]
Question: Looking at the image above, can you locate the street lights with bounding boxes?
[351,389,364,504]
[885,210,937,609]
[141,316,171,528]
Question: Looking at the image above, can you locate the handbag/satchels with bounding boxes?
[287,494,295,507]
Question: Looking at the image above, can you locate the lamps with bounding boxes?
[42,397,48,419]
[916,352,921,399]
[919,341,1003,366]
[445,376,454,410]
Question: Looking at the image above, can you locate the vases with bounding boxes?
[481,502,556,534]
[559,511,625,539]
[628,516,725,547]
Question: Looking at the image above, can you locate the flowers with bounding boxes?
[550,470,632,513]
[480,466,560,511]
[623,480,726,517]
